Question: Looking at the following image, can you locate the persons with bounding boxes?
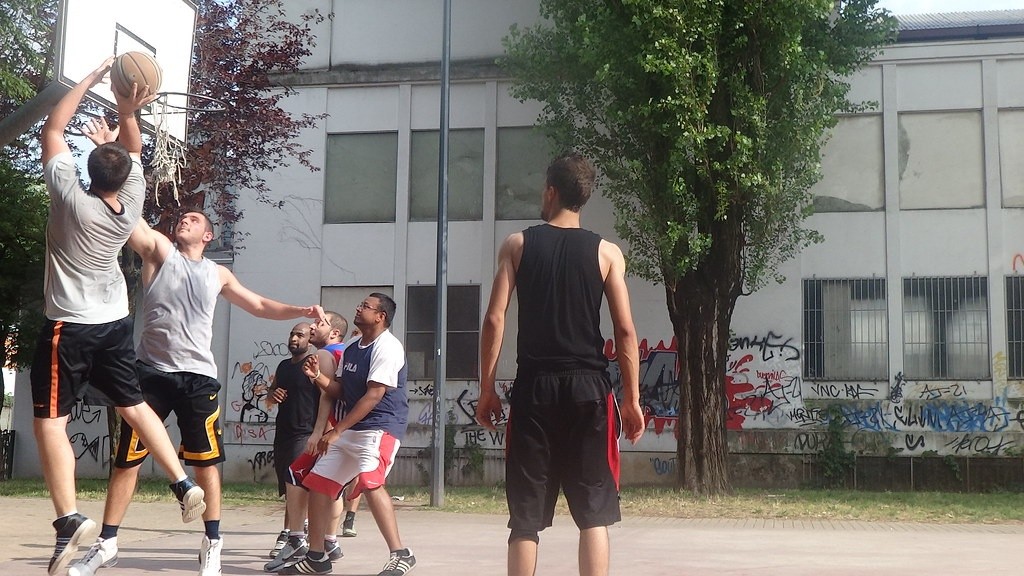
[265,293,417,576]
[476,152,646,576]
[67,116,331,576]
[28,55,208,576]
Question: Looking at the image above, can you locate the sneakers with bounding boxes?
[340,517,357,537]
[377,547,418,576]
[198,534,223,576]
[278,549,333,575]
[324,540,344,563]
[169,476,207,524]
[66,535,119,576]
[264,518,310,573]
[48,513,97,576]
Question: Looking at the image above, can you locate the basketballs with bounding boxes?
[110,51,163,99]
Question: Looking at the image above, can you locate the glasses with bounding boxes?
[357,304,380,312]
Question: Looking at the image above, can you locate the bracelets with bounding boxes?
[310,370,321,379]
